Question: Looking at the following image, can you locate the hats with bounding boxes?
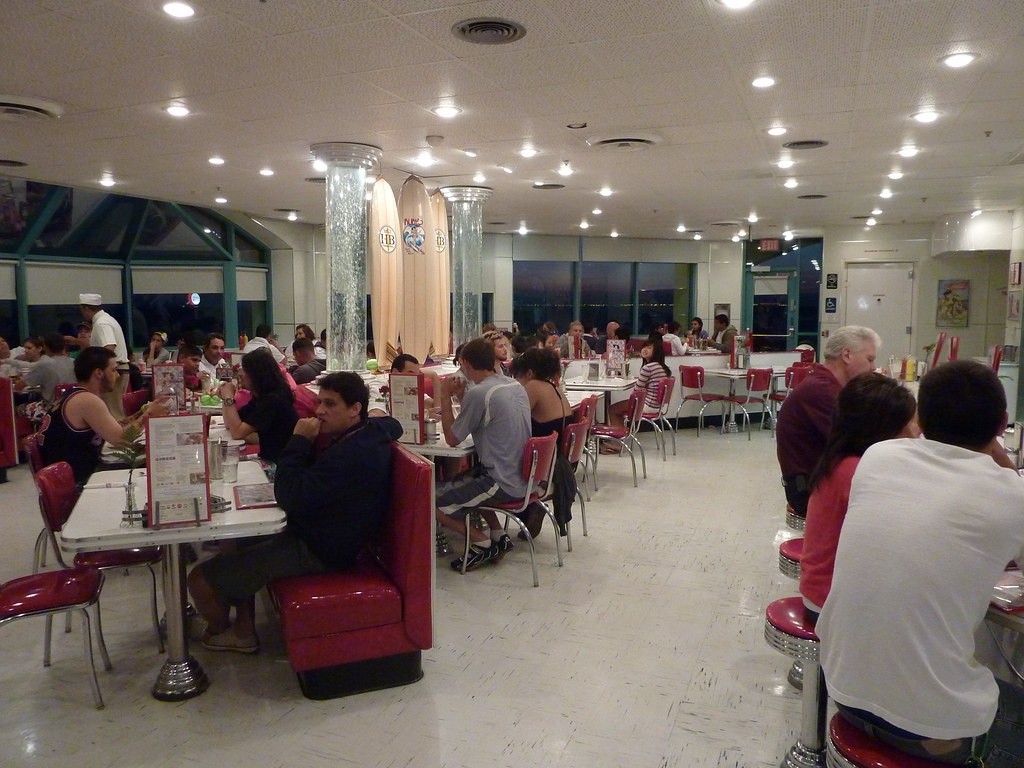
[74,321,91,331]
[155,328,168,343]
[79,293,102,306]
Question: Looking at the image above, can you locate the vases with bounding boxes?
[118,482,144,530]
[559,380,565,394]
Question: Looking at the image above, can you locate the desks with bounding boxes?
[62,460,288,701]
[703,368,748,433]
[564,391,604,409]
[752,366,787,430]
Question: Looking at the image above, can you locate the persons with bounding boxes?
[0,292,738,652]
[813,359,1024,768]
[798,371,922,624]
[775,325,881,520]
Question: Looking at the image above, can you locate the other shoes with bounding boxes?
[518,503,548,540]
[201,631,258,653]
[188,613,213,640]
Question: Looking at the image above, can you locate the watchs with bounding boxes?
[224,397,236,406]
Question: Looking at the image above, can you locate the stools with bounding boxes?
[779,537,805,691]
[825,712,970,767]
[786,503,806,532]
[763,596,828,768]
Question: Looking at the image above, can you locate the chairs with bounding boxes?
[674,365,727,435]
[653,375,676,449]
[619,378,677,460]
[580,388,647,487]
[35,461,164,651]
[760,360,818,430]
[575,394,598,501]
[723,367,775,440]
[507,415,592,551]
[460,431,564,588]
[0,568,110,710]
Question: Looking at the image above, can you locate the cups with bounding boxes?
[689,336,708,350]
[201,379,210,393]
[140,363,146,372]
[220,445,240,483]
[207,440,228,480]
[620,361,629,379]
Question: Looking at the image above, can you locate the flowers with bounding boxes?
[921,343,935,373]
[380,385,390,412]
[562,361,571,379]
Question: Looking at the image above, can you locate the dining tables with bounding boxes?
[564,375,639,441]
[399,422,475,555]
[101,413,257,458]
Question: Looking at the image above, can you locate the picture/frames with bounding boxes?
[936,278,970,327]
[1006,289,1022,320]
[1008,261,1021,285]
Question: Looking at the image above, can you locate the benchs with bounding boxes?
[258,387,435,702]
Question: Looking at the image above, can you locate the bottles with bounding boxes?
[425,418,437,445]
[240,334,248,350]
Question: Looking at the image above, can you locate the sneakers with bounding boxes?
[450,543,501,571]
[490,534,515,557]
